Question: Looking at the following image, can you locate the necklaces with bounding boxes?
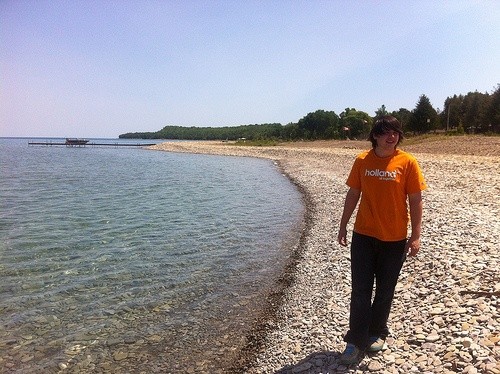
[375,147,392,157]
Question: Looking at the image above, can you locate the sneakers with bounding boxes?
[340,342,360,365]
[368,336,387,352]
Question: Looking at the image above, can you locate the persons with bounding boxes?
[336,116,428,364]
